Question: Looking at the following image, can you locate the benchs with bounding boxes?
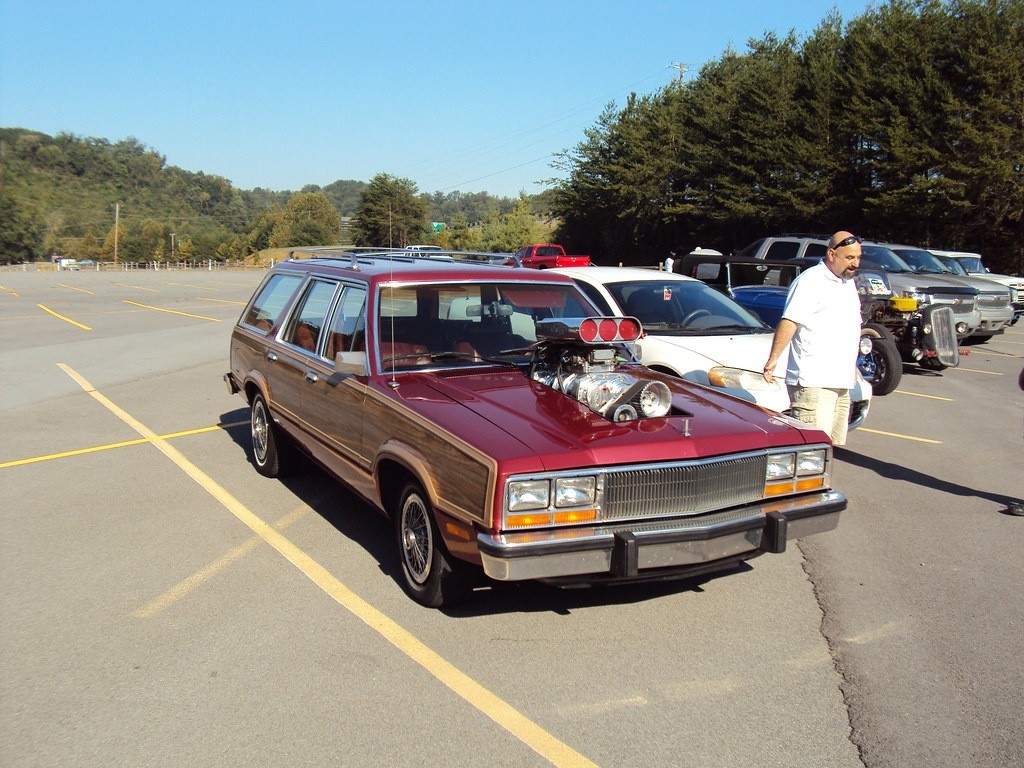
[297,316,450,353]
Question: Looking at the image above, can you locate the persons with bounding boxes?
[1007,366,1024,516]
[286,251,318,259]
[763,231,862,446]
[664,252,676,272]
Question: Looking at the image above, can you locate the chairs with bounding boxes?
[453,321,529,363]
[326,314,430,375]
[627,288,674,325]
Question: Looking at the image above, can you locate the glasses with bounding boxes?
[833,236,863,250]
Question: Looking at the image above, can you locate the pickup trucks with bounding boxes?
[503,244,590,272]
[403,244,455,259]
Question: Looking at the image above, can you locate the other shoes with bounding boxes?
[1008,502,1024,516]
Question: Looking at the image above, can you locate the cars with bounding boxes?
[226,250,842,611]
[670,236,1024,395]
[537,264,873,435]
